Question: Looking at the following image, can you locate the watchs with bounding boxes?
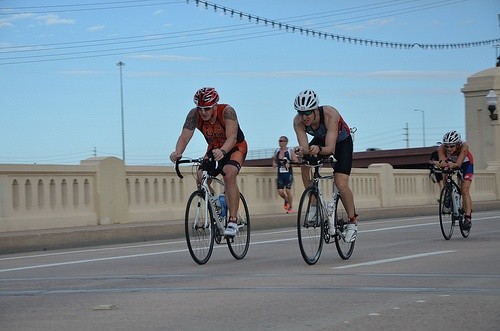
[318,146,322,154]
[220,149,226,155]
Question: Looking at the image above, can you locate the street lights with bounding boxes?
[116,61,126,161]
[414,109,425,146]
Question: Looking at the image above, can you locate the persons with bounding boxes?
[273,136,297,213]
[291,88,358,243]
[429,142,443,190]
[438,131,474,231]
[169,87,248,235]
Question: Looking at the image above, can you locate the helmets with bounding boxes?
[443,131,460,144]
[193,87,220,108]
[293,89,319,111]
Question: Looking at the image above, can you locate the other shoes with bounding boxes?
[287,209,294,214]
[284,199,289,209]
[464,215,472,227]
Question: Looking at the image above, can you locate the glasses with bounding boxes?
[279,140,285,142]
[297,111,313,116]
[445,145,455,149]
[196,106,213,111]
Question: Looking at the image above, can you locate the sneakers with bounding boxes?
[308,206,318,222]
[223,223,238,235]
[345,222,358,243]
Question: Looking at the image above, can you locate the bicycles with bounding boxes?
[175,149,250,265]
[429,159,473,240]
[273,150,359,265]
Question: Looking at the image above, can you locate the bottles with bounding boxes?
[219,193,227,217]
[455,192,459,209]
[212,193,221,215]
